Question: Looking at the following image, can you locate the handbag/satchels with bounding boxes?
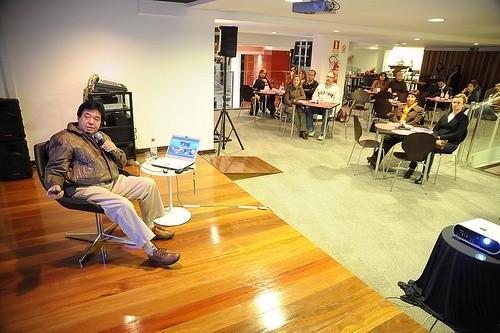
[336,109,346,122]
[250,102,259,116]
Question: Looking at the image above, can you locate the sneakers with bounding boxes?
[148,245,179,265]
[151,225,174,240]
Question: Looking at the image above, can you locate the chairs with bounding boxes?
[34,142,137,267]
[238,85,500,195]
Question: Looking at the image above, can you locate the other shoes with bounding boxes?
[367,155,376,167]
[271,115,278,120]
[318,132,324,141]
[299,131,308,139]
[309,129,315,137]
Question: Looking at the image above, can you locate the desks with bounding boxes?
[259,91,285,120]
[290,100,340,144]
[398,224,500,333]
[373,123,433,184]
[405,81,426,91]
[346,76,363,93]
[141,157,196,226]
[426,97,453,131]
[366,90,378,101]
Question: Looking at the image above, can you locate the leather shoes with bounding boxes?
[414,175,428,184]
[404,169,414,179]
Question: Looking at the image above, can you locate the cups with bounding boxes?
[145,151,153,165]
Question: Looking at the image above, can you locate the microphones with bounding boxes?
[94,134,118,158]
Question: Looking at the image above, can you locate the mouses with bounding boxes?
[175,168,182,173]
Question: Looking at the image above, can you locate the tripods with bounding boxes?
[214,56,244,155]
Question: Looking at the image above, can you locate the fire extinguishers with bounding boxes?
[329,56,341,84]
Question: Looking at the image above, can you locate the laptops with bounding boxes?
[151,136,202,171]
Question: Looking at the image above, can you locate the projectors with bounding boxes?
[453,218,500,256]
[291,0,337,13]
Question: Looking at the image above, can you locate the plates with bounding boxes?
[375,123,434,136]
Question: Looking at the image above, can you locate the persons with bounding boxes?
[253,67,339,142]
[403,94,470,184]
[43,99,181,266]
[366,90,424,170]
[370,63,500,112]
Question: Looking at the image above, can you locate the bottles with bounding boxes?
[150,138,157,160]
[440,90,449,100]
[315,91,320,103]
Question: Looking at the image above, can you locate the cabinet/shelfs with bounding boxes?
[83,91,136,161]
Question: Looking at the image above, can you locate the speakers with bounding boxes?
[0,99,26,140]
[219,26,238,57]
[0,142,33,180]
